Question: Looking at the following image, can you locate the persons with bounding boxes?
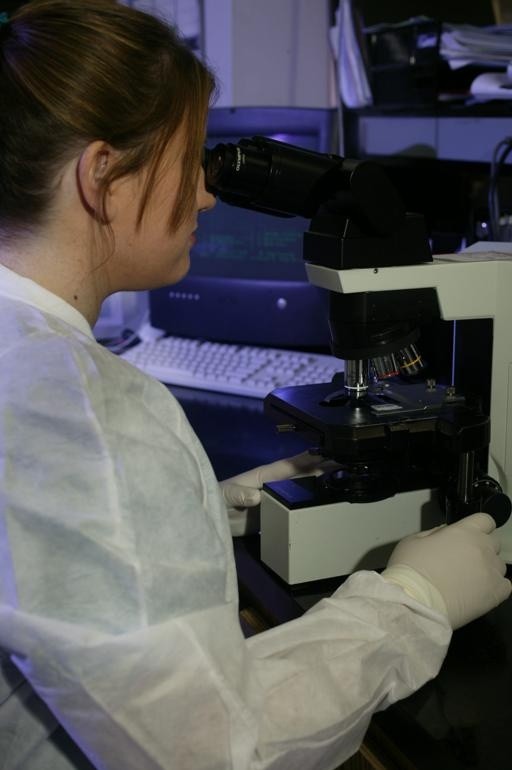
[2,1,511,770]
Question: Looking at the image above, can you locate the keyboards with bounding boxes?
[119,335,345,400]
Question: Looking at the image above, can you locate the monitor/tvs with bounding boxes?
[149,105,339,346]
